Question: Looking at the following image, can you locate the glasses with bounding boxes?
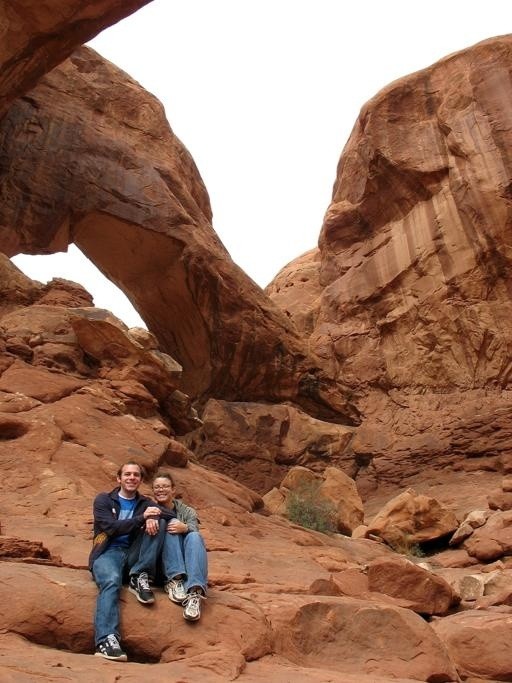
[152,484,174,491]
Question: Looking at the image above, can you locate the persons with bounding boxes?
[86,457,179,663]
[152,471,210,626]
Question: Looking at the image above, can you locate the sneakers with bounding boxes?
[182,589,203,622]
[128,570,156,605]
[164,578,187,603]
[93,634,128,663]
